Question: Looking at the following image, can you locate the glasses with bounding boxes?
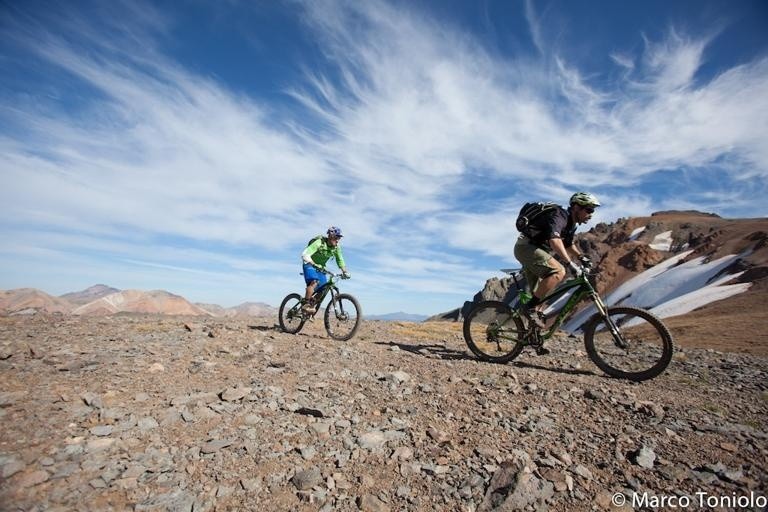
[584,207,594,214]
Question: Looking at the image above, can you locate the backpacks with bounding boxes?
[515,201,576,242]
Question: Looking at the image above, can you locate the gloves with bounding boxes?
[566,261,582,278]
[576,254,592,268]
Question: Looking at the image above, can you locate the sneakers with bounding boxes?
[532,344,550,354]
[521,303,545,329]
[300,304,316,313]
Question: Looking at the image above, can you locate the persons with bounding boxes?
[511,190,604,356]
[300,226,352,314]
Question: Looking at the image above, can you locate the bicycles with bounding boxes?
[462,254,675,382]
[278,269,363,342]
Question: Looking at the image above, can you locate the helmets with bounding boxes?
[569,192,600,207]
[326,227,342,237]
